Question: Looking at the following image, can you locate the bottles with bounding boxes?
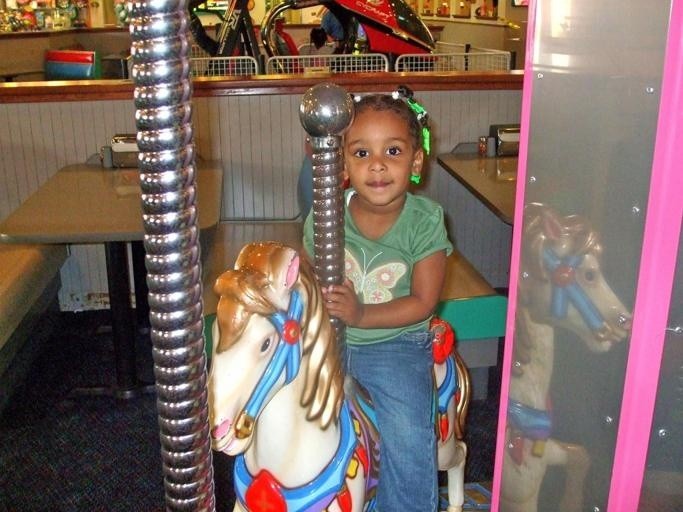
[479,136,497,159]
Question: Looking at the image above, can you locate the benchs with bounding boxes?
[198,135,508,402]
[0,242,70,408]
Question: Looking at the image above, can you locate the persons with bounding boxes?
[309,28,342,70]
[302,86,453,511]
[322,6,358,44]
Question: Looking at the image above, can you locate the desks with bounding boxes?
[436,149,516,225]
[0,163,224,402]
